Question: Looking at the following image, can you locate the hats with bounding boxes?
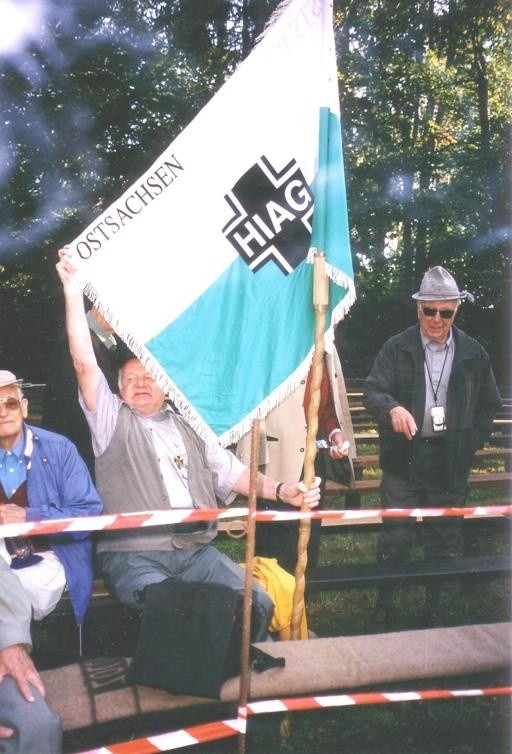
[411,265,467,301]
[0,370,23,387]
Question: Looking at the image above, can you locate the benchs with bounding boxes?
[0,371,512,754]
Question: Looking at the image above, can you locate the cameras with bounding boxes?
[430,406,447,432]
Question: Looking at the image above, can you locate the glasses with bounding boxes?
[0,397,21,410]
[420,303,457,318]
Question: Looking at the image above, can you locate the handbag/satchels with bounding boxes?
[125,577,238,700]
[238,556,307,640]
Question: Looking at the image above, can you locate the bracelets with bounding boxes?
[272,481,286,506]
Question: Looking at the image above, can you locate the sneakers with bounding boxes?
[366,608,388,634]
[422,610,445,629]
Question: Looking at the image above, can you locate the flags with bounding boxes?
[58,0,362,454]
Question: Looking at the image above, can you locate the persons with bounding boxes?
[0,367,103,657]
[358,262,504,636]
[0,555,65,753]
[231,339,361,652]
[53,241,321,649]
